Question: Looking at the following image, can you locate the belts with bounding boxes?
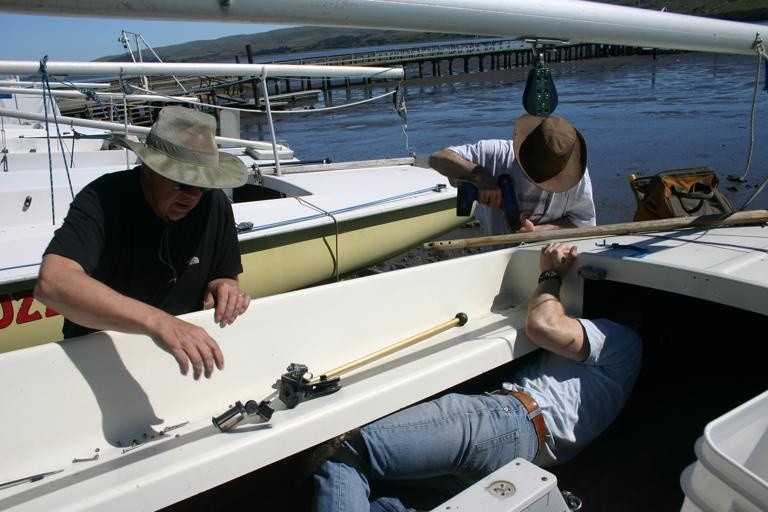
[499,385,547,464]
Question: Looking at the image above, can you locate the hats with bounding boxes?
[511,112,589,195]
[110,104,250,191]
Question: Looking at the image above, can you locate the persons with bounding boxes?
[32,105,250,380]
[315,241,654,512]
[429,114,596,252]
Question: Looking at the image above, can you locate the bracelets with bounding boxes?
[538,271,563,285]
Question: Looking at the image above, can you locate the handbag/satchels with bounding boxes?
[628,165,738,224]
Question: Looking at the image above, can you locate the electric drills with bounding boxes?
[456,173,522,233]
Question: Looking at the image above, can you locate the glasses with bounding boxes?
[168,179,214,194]
